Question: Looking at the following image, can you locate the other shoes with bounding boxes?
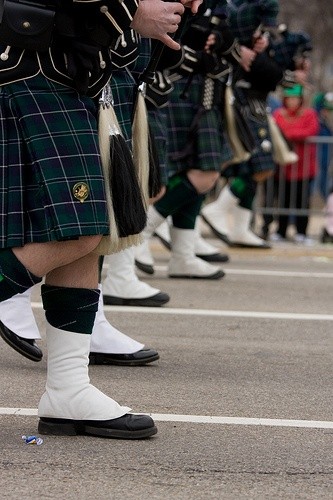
[103,290,170,307]
[152,232,171,252]
[195,251,230,262]
[169,269,225,279]
[38,413,158,439]
[232,240,272,249]
[88,347,159,366]
[200,213,232,246]
[0,320,43,362]
[135,258,154,275]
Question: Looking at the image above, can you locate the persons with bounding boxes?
[0,0,333,367]
[0,0,185,439]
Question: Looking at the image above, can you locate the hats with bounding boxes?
[282,84,303,97]
